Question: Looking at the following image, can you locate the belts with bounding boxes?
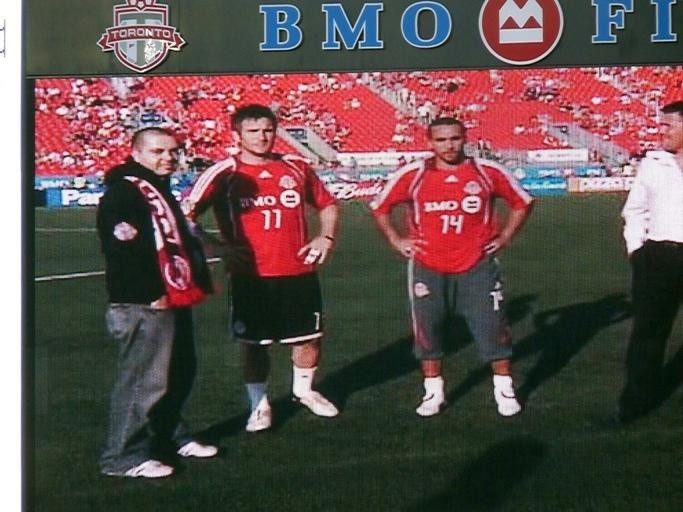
[644,240,682,248]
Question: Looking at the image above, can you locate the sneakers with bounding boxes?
[291,390,340,418]
[245,403,273,433]
[177,441,218,458]
[415,393,445,418]
[493,386,522,417]
[100,459,174,479]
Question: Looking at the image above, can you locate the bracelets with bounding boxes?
[321,232,335,242]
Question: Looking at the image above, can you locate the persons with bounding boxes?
[610,101,683,424]
[177,104,341,434]
[367,117,535,418]
[32,64,681,182]
[92,126,220,484]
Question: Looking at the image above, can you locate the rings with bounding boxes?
[405,247,412,254]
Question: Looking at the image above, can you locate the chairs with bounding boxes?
[34,66,683,181]
